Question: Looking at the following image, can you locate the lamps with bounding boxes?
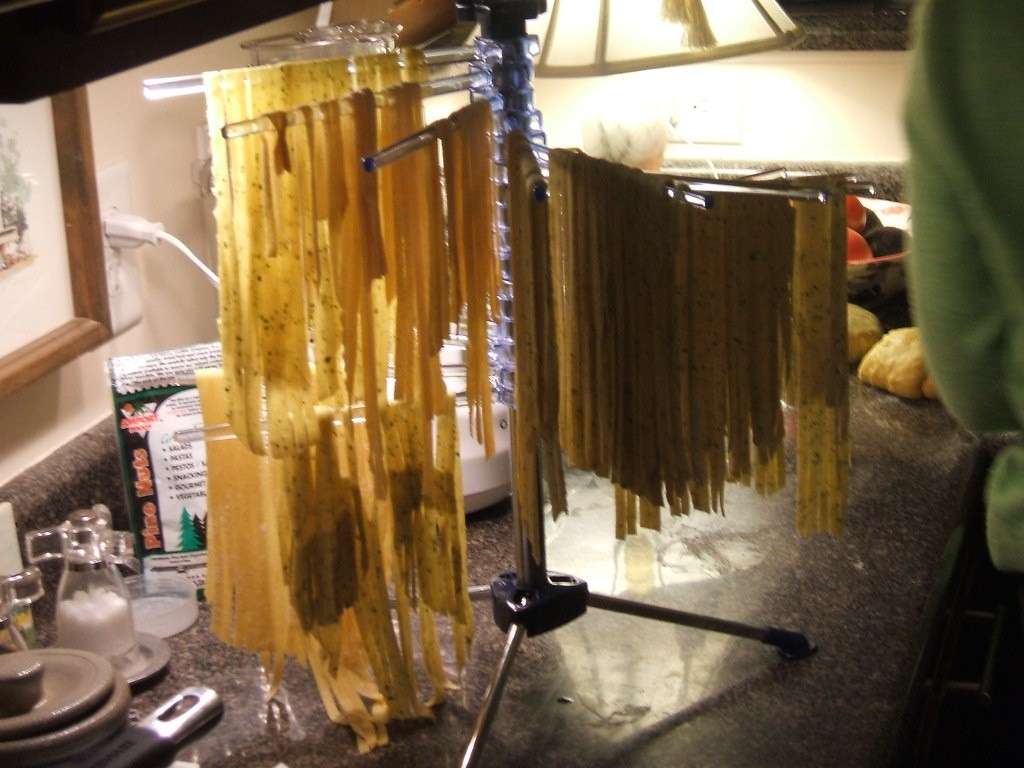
[526,0,808,78]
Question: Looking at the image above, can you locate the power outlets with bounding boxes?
[87,156,145,354]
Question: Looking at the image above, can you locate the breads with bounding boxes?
[846,302,943,400]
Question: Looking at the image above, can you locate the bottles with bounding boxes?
[57,513,133,677]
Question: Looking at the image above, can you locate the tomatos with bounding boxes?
[845,193,872,264]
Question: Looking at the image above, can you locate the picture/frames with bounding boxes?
[0,85,114,402]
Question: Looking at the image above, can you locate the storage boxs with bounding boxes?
[110,341,268,604]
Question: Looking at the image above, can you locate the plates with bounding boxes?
[51,631,172,684]
